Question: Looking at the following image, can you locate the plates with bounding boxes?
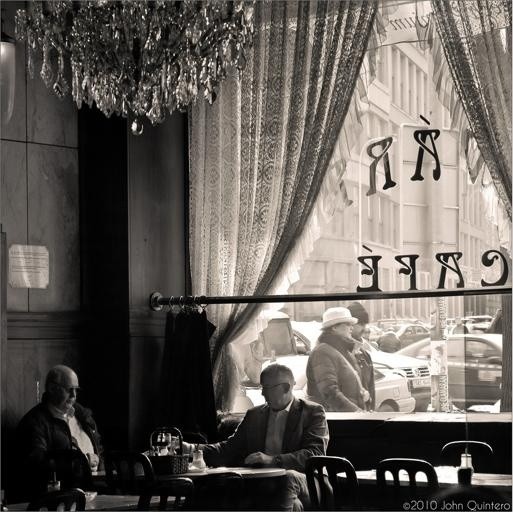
[188,468,204,473]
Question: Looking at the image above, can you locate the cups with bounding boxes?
[458,468,471,485]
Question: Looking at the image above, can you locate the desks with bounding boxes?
[336,466,512,510]
[1,494,184,511]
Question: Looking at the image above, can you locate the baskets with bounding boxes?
[142,427,190,476]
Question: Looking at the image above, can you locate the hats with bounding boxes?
[347,302,368,324]
[320,307,358,331]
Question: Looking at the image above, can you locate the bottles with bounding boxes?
[193,449,206,469]
[460,453,474,476]
[157,432,179,454]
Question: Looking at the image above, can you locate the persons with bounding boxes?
[344,302,377,411]
[361,327,371,343]
[452,317,469,334]
[376,327,403,353]
[17,364,129,495]
[306,307,372,413]
[176,363,335,511]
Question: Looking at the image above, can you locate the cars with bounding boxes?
[289,320,431,408]
[392,333,501,410]
[371,324,430,347]
[243,355,415,412]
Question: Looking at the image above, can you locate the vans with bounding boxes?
[447,317,475,326]
[466,314,491,329]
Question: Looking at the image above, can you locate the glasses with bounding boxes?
[258,383,286,390]
[66,386,81,394]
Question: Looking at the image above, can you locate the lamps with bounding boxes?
[11,1,255,137]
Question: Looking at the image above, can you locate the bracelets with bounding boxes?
[271,453,281,468]
